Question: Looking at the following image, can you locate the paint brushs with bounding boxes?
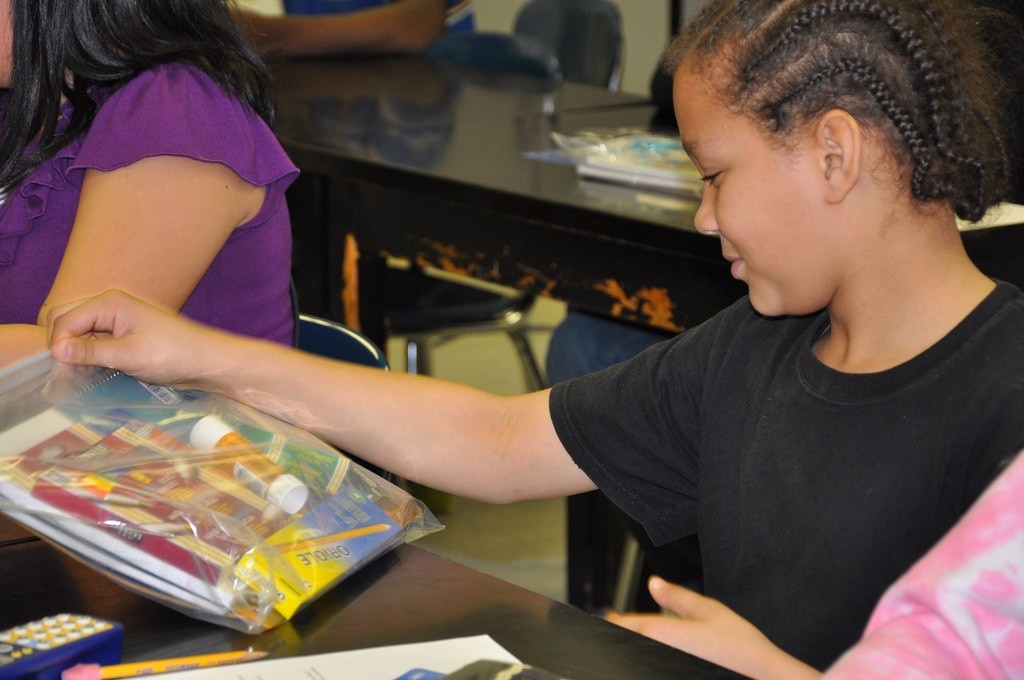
[63,486,199,536]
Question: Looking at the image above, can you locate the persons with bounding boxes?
[226,0,474,66]
[1,0,300,407]
[46,0,1024,680]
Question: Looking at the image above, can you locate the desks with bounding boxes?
[277,104,1023,354]
[0,538,749,680]
[271,56,649,317]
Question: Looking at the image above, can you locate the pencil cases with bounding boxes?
[0,611,125,680]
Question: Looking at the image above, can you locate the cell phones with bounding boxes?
[0,613,124,680]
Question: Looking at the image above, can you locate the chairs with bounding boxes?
[512,0,624,90]
[296,311,390,371]
[381,31,564,393]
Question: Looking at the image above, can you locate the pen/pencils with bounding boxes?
[260,523,393,557]
[60,648,273,680]
